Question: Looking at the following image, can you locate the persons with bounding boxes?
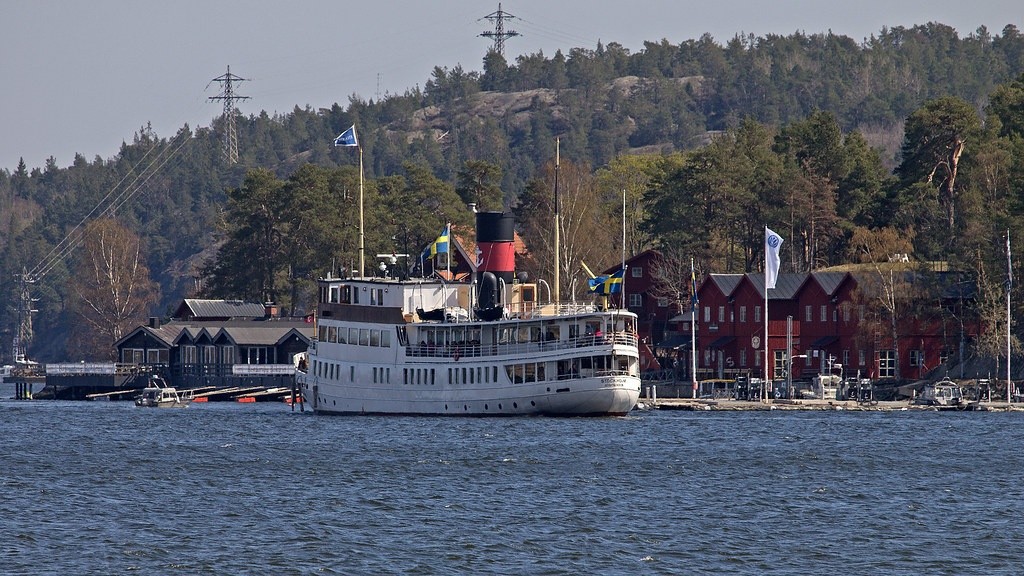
[537,328,544,352]
[421,340,480,357]
[299,356,306,372]
[558,364,577,380]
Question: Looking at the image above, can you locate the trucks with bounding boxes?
[802,376,846,401]
[835,368,872,401]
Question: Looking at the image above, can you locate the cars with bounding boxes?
[796,389,810,399]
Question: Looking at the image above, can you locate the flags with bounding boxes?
[303,313,314,323]
[423,225,448,260]
[333,125,357,147]
[588,269,625,296]
[765,227,783,290]
[690,272,699,311]
[1006,242,1013,292]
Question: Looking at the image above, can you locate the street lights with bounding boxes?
[788,354,807,399]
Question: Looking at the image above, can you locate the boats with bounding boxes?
[134,375,195,407]
[913,376,977,411]
[293,121,641,416]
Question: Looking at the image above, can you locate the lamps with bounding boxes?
[389,257,397,280]
[379,262,387,278]
